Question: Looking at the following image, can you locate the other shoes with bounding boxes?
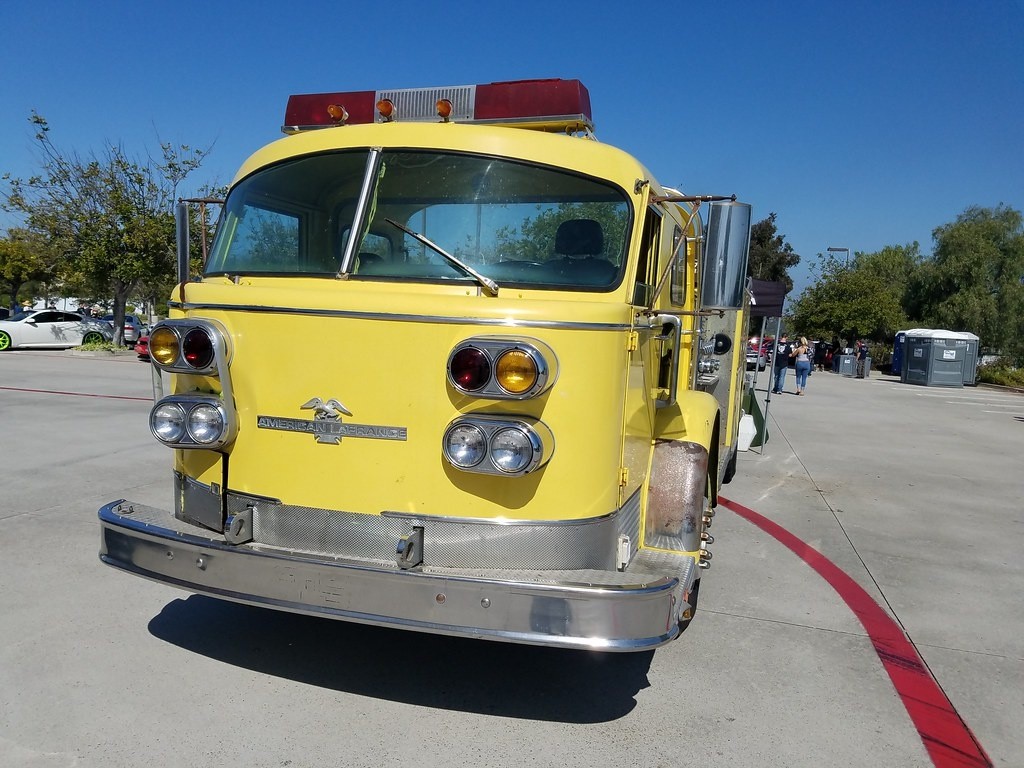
[856,377,860,378]
[861,377,864,378]
[777,392,781,394]
[795,390,801,395]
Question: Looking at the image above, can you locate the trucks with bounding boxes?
[98,77,756,657]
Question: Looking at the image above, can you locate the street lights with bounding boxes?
[828,247,851,270]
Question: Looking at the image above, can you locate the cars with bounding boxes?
[100,314,150,344]
[134,336,149,356]
[750,337,778,361]
[0,309,114,351]
[746,347,766,370]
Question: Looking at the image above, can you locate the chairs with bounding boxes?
[355,253,384,274]
[541,218,617,284]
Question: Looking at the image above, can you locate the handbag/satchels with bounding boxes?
[807,347,815,358]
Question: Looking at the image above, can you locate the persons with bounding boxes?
[814,336,868,379]
[14,296,114,320]
[771,332,816,394]
